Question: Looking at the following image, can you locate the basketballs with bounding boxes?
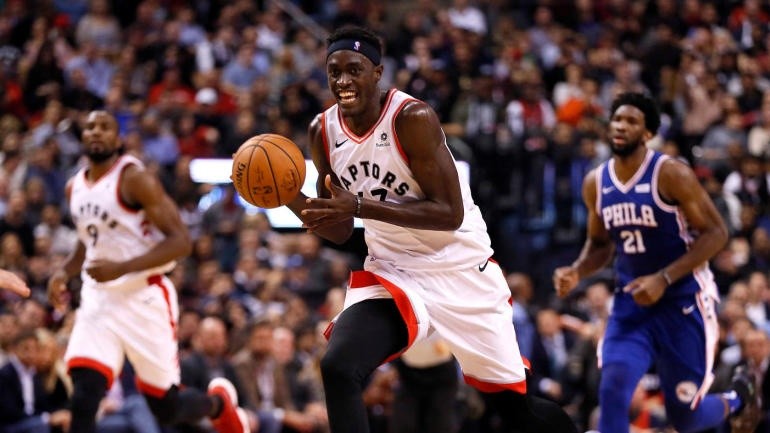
[231,132,307,209]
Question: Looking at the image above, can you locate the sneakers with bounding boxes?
[729,366,762,433]
[207,377,250,433]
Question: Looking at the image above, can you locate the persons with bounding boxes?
[0,0,770,433]
